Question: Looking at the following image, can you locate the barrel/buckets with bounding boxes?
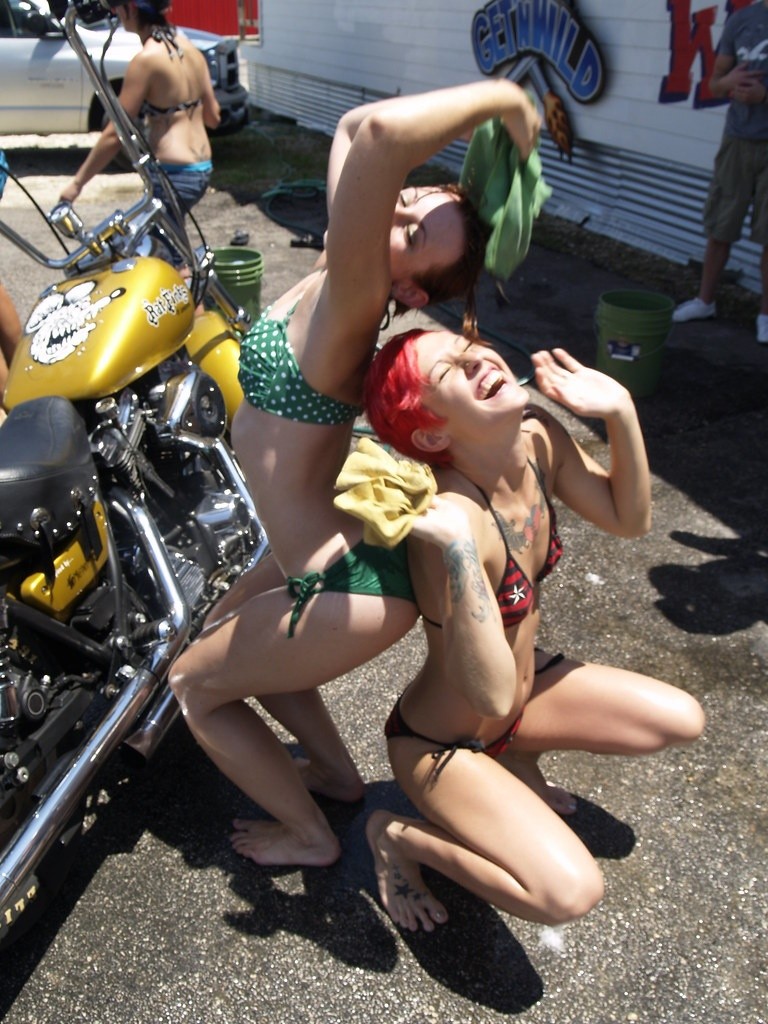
[201,248,263,322]
[592,290,674,399]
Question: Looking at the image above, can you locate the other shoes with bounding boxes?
[672,296,717,323]
[756,313,768,344]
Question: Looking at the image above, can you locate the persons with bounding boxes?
[674,0,768,341]
[60,0,221,268]
[0,283,24,417]
[367,327,706,932]
[168,79,540,865]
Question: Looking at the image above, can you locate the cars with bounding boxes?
[0,0,253,136]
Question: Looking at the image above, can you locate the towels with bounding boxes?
[334,438,437,552]
[460,116,552,279]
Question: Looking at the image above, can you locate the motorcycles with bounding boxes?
[0,1,278,960]
